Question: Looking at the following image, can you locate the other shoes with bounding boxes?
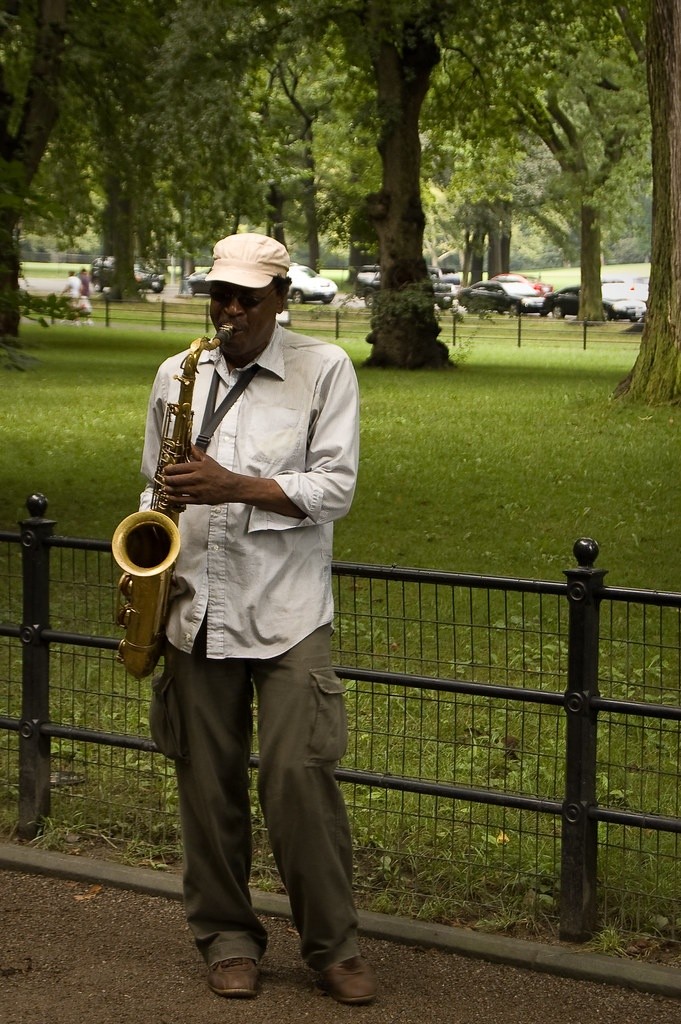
[207,957,260,997]
[320,956,377,1005]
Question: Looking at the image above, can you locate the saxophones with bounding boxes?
[109,323,239,680]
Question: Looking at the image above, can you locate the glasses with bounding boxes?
[210,285,278,308]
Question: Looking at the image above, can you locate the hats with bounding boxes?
[204,234,290,288]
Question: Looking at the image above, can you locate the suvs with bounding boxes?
[599,276,650,302]
[90,256,165,293]
[355,264,456,309]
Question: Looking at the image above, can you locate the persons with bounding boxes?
[61,269,93,325]
[138,233,383,1001]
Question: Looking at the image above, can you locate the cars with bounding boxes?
[286,262,338,304]
[185,270,212,295]
[456,279,546,316]
[541,285,647,321]
[488,273,554,297]
